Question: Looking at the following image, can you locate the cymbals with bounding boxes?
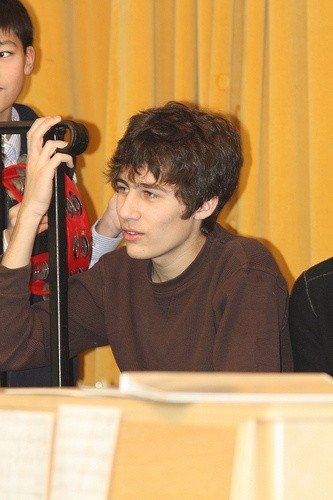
[3,153,94,298]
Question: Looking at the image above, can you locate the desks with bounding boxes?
[0,388,332,500]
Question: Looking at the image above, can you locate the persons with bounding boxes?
[0,101,293,374]
[0,0,124,389]
